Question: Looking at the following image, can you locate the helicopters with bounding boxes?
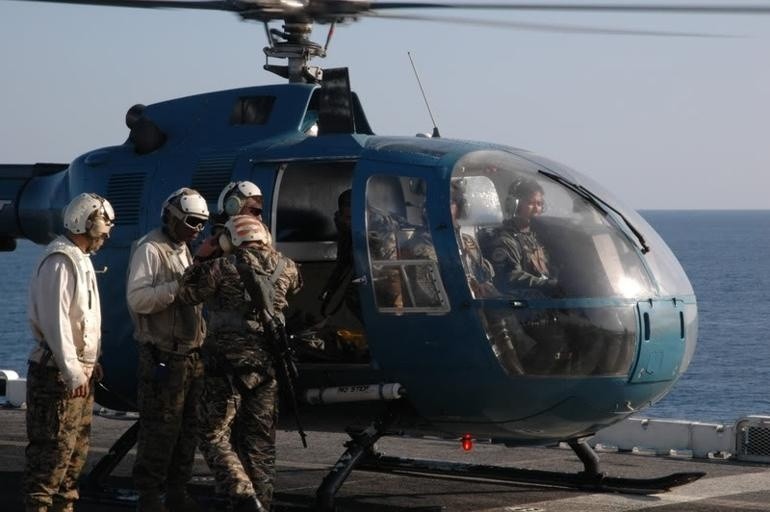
[0,1,770,510]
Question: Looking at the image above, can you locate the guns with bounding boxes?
[235,261,310,447]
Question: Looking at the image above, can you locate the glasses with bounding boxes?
[249,206,263,215]
[107,223,115,238]
[184,213,209,231]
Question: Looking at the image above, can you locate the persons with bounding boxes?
[328,190,400,319]
[402,179,523,376]
[489,179,597,341]
[18,193,116,512]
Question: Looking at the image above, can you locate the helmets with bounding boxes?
[219,215,273,252]
[217,180,263,215]
[63,192,115,238]
[160,187,210,224]
